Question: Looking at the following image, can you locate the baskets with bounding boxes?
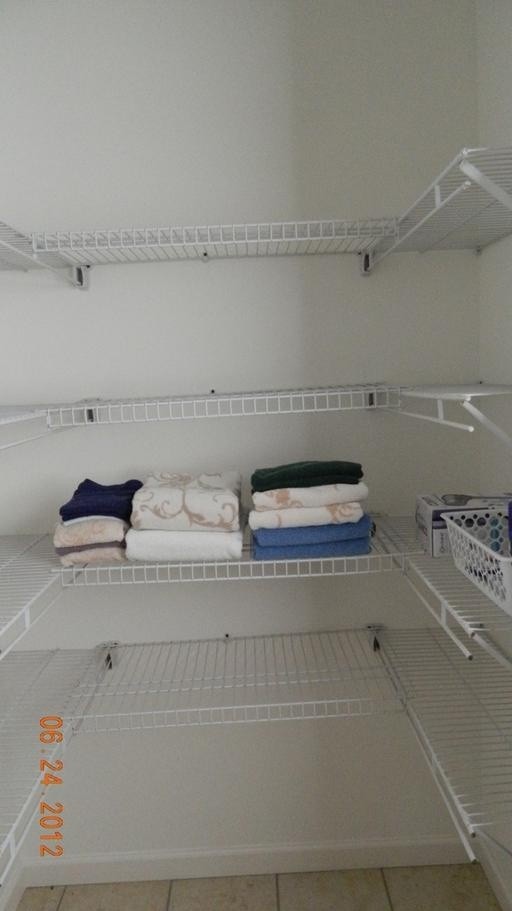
[441,504,512,620]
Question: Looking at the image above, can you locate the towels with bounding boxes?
[53,515,129,568]
[59,478,143,523]
[130,471,244,532]
[123,528,243,565]
[248,460,373,561]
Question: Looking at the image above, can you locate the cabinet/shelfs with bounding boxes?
[32,215,404,737]
[365,142,512,867]
[1,217,108,899]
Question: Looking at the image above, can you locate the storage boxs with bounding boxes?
[414,489,512,558]
